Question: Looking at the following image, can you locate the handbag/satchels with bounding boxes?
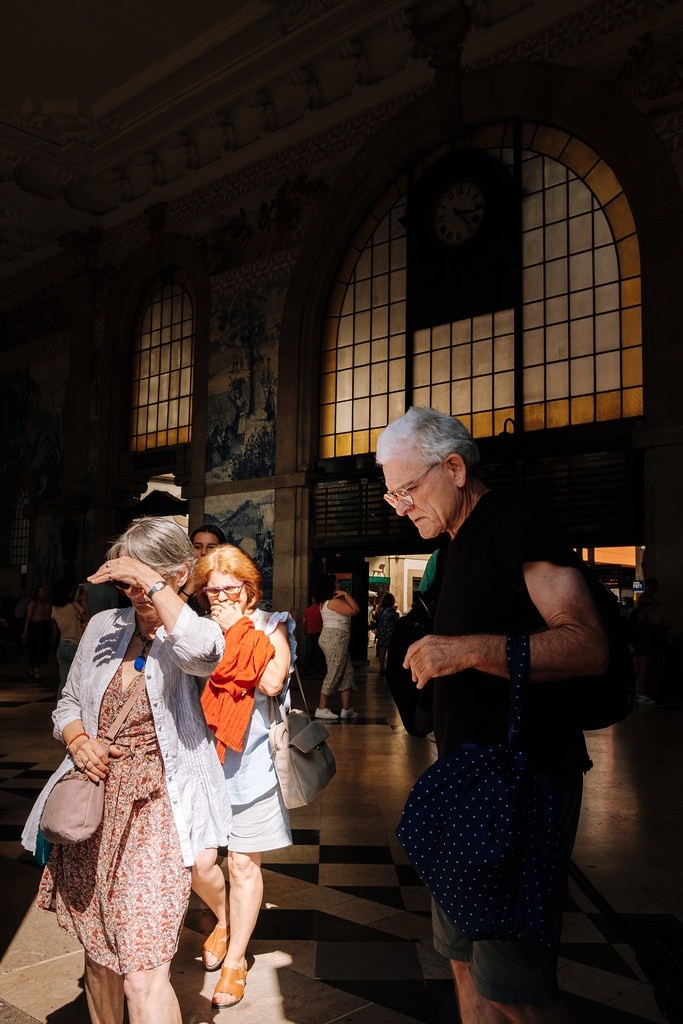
[40,770,105,843]
[268,708,337,810]
[396,632,564,947]
[385,583,435,739]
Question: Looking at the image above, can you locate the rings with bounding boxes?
[217,612,220,617]
[108,573,112,579]
[219,607,223,611]
[105,561,111,569]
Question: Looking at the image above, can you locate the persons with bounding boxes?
[303,572,361,719]
[375,593,398,675]
[49,579,89,703]
[376,406,610,1024]
[21,517,232,1024]
[176,524,229,617]
[82,567,118,617]
[620,577,672,704]
[187,544,299,1008]
[21,584,53,683]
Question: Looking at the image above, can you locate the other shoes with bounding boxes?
[340,707,359,718]
[633,694,639,703]
[637,695,656,704]
[315,708,338,719]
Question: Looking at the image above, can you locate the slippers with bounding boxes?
[202,922,230,970]
[211,959,248,1008]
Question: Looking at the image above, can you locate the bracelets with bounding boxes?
[182,589,190,597]
[343,592,349,597]
[66,732,89,750]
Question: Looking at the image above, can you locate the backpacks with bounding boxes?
[497,502,635,732]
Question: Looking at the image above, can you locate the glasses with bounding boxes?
[113,579,131,590]
[383,460,440,508]
[203,579,246,596]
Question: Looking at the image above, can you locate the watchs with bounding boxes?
[148,580,169,599]
